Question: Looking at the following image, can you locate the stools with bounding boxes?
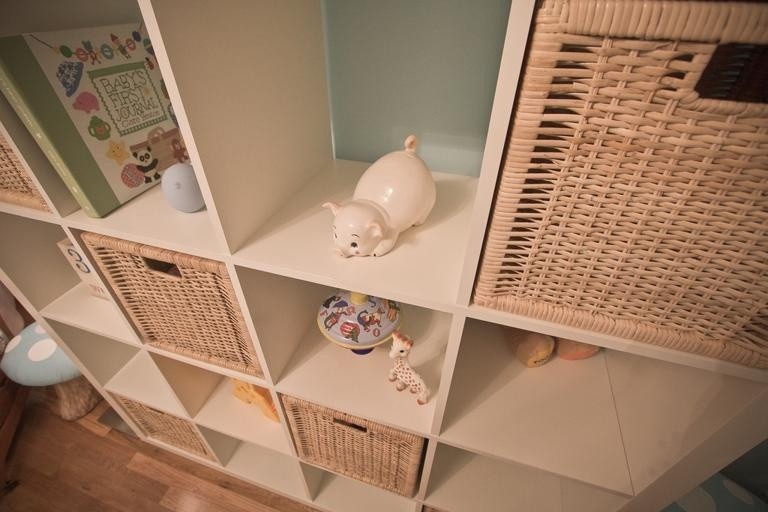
[0,320,104,423]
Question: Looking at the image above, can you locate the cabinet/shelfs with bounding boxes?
[0,1,768,512]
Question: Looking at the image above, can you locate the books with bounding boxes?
[0,20,192,219]
